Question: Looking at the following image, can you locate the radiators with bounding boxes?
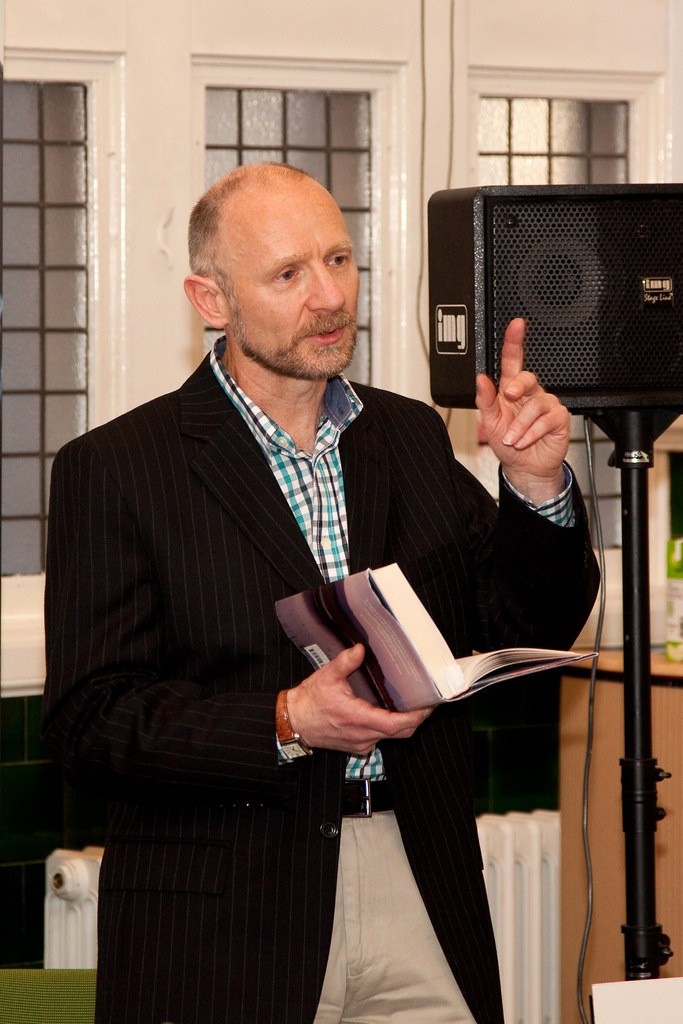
[42,807,562,1024]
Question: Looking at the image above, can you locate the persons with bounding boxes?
[42,161,603,1024]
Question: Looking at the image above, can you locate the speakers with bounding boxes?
[428,183,682,410]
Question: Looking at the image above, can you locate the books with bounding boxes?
[274,562,598,716]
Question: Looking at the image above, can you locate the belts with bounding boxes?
[339,777,394,818]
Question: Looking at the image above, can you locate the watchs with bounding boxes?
[275,689,313,761]
